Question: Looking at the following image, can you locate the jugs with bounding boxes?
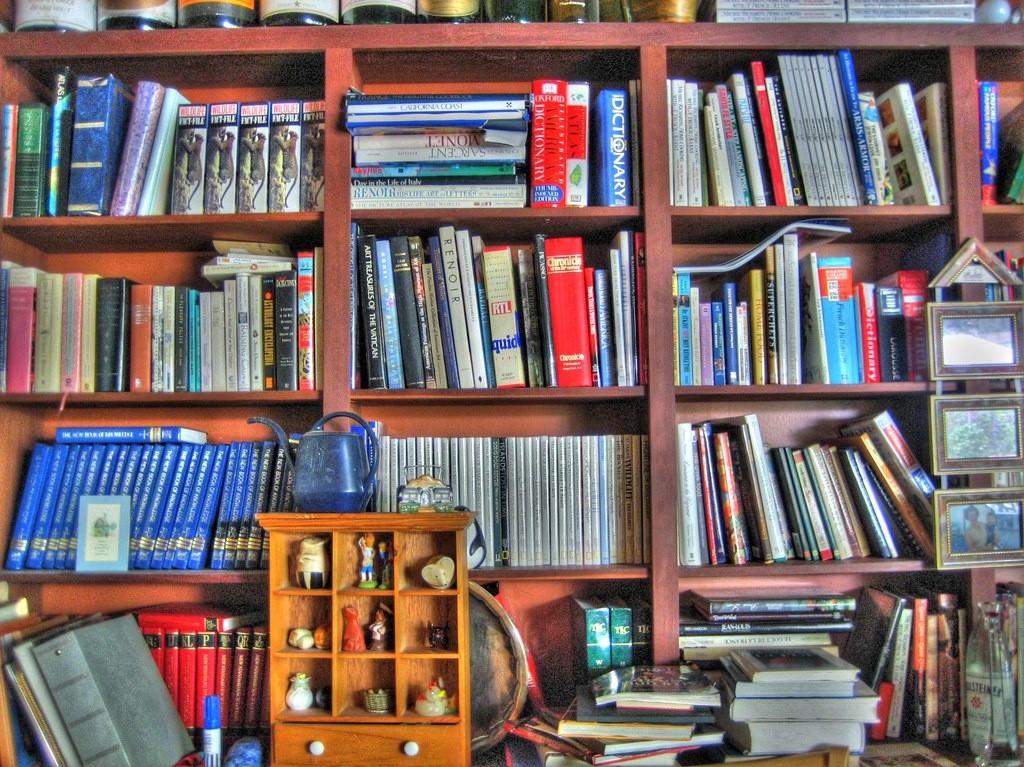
[245,410,381,512]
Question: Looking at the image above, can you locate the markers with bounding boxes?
[202,695,223,767]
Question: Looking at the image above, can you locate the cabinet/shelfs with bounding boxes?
[2,0,1024,765]
[250,511,474,767]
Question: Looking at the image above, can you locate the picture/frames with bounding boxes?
[924,301,1023,381]
[928,392,1024,476]
[932,487,1023,570]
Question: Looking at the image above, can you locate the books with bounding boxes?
[978,81,1000,207]
[0,224,1022,396]
[1000,100,1024,204]
[698,0,977,24]
[4,425,1022,570]
[0,583,1022,767]
[2,48,955,217]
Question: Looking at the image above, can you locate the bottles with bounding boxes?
[967,598,1017,757]
